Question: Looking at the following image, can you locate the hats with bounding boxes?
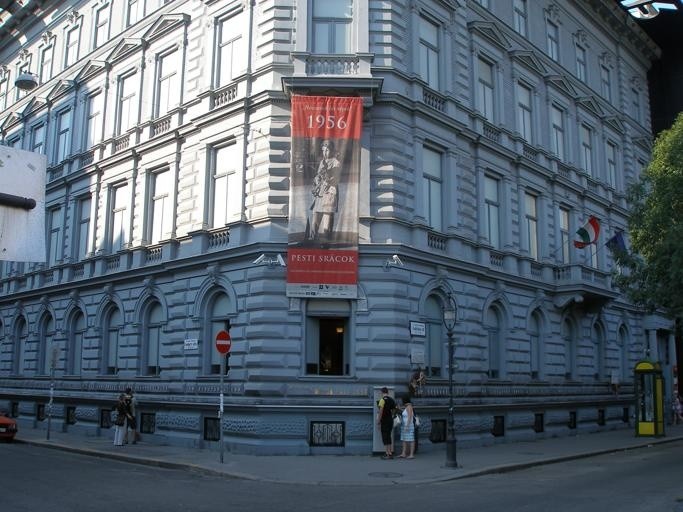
[119,393,131,398]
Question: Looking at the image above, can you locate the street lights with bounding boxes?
[441,291,457,468]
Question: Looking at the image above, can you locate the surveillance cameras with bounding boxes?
[252,254,266,265]
[277,254,286,267]
[393,255,403,264]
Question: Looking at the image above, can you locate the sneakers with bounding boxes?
[382,453,415,459]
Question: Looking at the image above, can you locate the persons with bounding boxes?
[376,387,396,459]
[308,138,342,242]
[113,393,133,446]
[125,388,137,444]
[671,364,678,391]
[397,394,416,459]
[671,390,683,425]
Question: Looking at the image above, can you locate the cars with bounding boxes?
[0,405,18,442]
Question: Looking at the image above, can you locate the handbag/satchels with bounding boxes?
[110,410,119,422]
[392,415,400,427]
[414,415,419,429]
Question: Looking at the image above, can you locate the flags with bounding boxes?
[572,215,601,249]
[606,233,625,250]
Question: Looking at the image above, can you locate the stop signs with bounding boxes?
[214,330,232,356]
